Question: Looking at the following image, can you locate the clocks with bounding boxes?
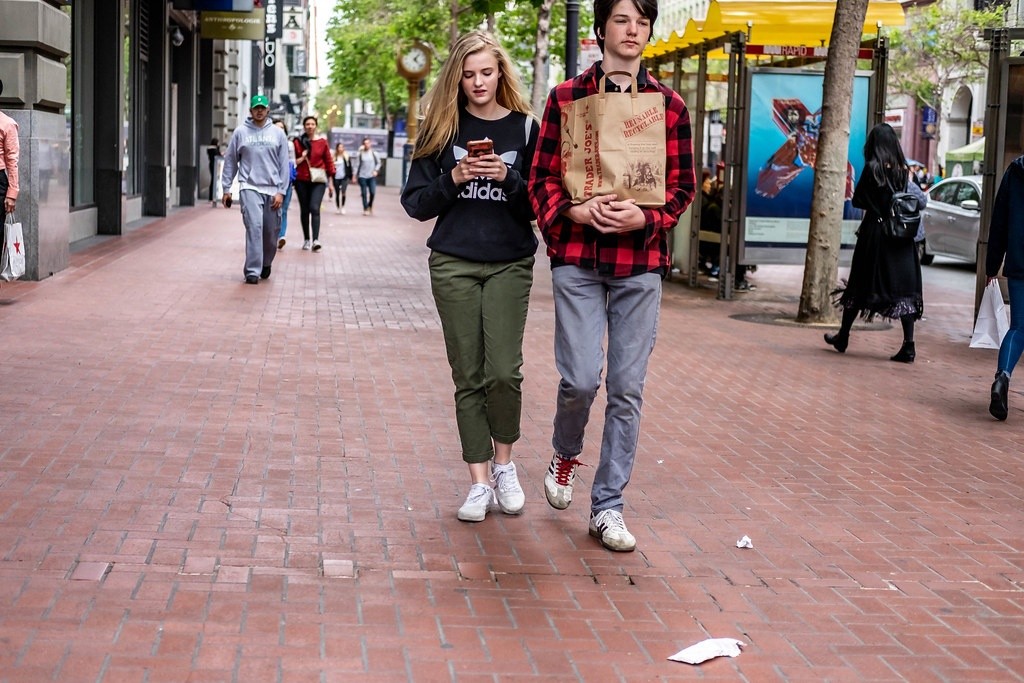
[402,47,427,72]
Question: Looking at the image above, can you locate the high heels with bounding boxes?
[988,369,1008,422]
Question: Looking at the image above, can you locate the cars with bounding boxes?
[915,175,984,266]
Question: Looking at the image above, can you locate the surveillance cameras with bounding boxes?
[172,28,183,46]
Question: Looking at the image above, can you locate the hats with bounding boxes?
[251,95,269,109]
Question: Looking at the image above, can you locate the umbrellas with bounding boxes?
[905,158,926,168]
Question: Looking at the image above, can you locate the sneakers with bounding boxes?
[589,509,636,551]
[488,454,526,514]
[544,449,589,510]
[458,483,498,521]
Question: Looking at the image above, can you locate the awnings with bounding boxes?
[946,136,986,161]
[592,1,908,62]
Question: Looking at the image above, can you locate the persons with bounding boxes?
[906,158,945,191]
[37,138,54,203]
[333,143,354,215]
[293,116,337,251]
[351,138,382,215]
[207,137,225,204]
[221,95,289,284]
[699,165,758,293]
[986,157,1023,419]
[0,77,20,280]
[526,1,697,552]
[270,118,297,249]
[399,30,541,521]
[823,122,923,362]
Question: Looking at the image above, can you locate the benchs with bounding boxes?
[699,229,730,244]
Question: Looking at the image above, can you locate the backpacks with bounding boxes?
[871,166,920,241]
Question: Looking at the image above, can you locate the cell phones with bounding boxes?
[467,140,493,164]
[226,193,232,208]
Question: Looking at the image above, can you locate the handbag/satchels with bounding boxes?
[560,70,666,209]
[969,277,1009,350]
[0,211,26,281]
[309,167,328,183]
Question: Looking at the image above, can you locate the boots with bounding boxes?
[824,330,849,353]
[891,340,914,362]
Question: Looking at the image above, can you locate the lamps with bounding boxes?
[165,23,184,46]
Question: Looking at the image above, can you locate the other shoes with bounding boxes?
[706,265,757,293]
[364,205,372,216]
[246,275,258,284]
[312,240,321,250]
[337,206,346,214]
[303,240,310,249]
[278,235,286,249]
[260,266,270,279]
